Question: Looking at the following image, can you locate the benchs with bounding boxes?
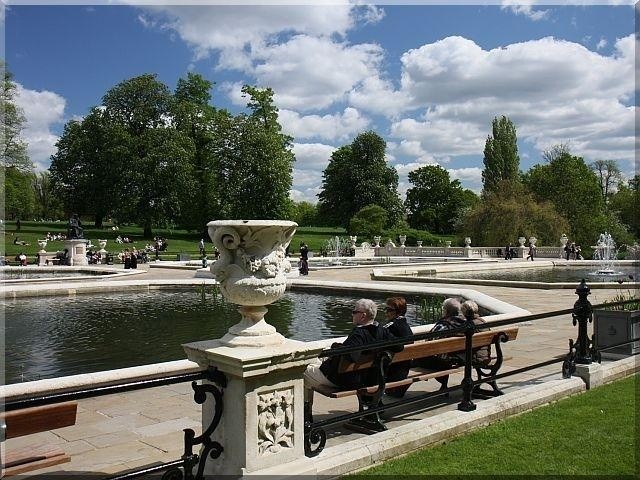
[310,326,519,436]
[0,399,78,479]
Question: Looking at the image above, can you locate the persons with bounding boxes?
[304,297,404,390]
[564,242,581,261]
[202,256,207,269]
[372,295,414,399]
[215,249,220,260]
[15,252,27,266]
[86,225,167,269]
[527,242,535,261]
[199,239,206,254]
[14,237,31,247]
[505,242,518,260]
[411,298,466,369]
[458,299,492,364]
[60,248,69,265]
[299,243,309,275]
[46,231,67,242]
